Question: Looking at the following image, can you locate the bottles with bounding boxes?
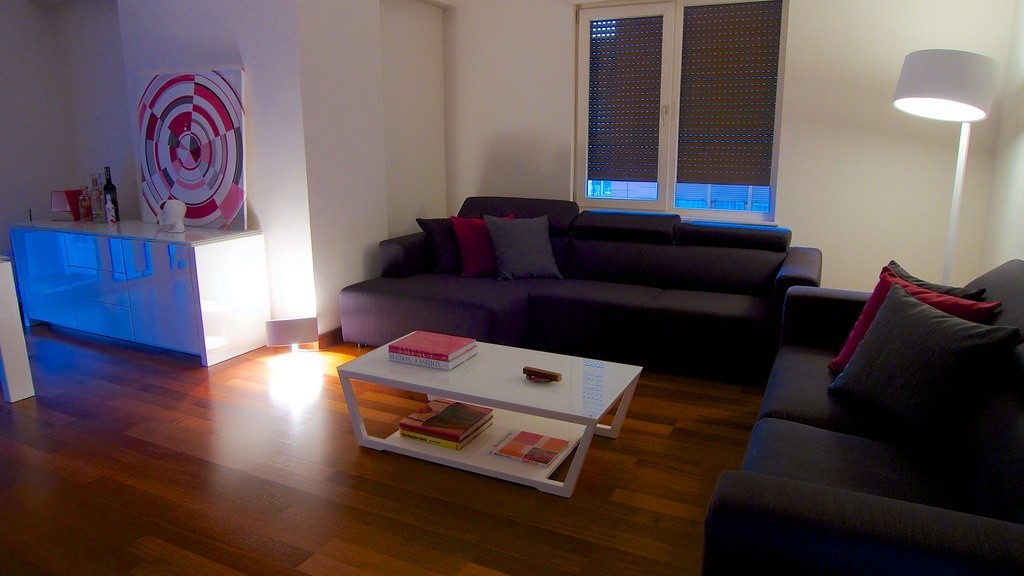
[104,166,120,223]
[105,193,116,223]
[78,186,94,223]
[90,172,106,222]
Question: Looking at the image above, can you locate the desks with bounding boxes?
[336,331,643,498]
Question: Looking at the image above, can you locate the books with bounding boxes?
[389,330,479,371]
[399,396,494,450]
[488,429,570,468]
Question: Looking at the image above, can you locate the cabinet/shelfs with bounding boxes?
[11,218,268,367]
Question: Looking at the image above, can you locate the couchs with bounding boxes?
[702,259,1024,576]
[339,197,823,363]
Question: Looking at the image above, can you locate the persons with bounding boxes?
[163,199,187,233]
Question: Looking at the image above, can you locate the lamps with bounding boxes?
[892,49,999,286]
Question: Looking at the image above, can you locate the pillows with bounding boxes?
[828,283,1023,432]
[828,266,1004,377]
[449,213,517,276]
[888,260,986,302]
[416,218,459,274]
[483,216,564,280]
